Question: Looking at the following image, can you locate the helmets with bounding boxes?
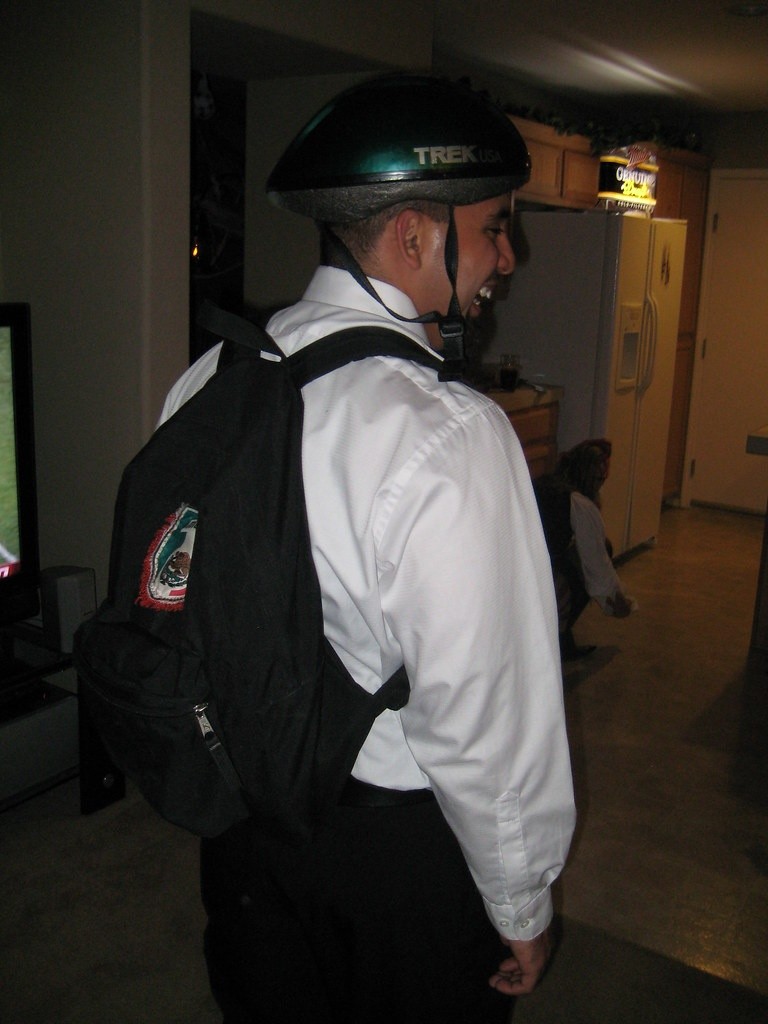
[262,74,531,224]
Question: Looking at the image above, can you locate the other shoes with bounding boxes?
[562,642,596,661]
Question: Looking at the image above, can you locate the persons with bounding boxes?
[151,75,577,1024]
[531,439,634,680]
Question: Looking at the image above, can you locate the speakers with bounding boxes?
[39,566,98,662]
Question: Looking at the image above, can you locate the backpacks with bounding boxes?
[74,302,442,843]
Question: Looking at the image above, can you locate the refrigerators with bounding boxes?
[481,207,689,562]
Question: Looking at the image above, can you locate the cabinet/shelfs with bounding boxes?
[484,114,712,501]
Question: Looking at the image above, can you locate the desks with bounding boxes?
[0,620,125,816]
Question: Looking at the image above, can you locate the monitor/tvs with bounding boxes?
[0,301,40,625]
